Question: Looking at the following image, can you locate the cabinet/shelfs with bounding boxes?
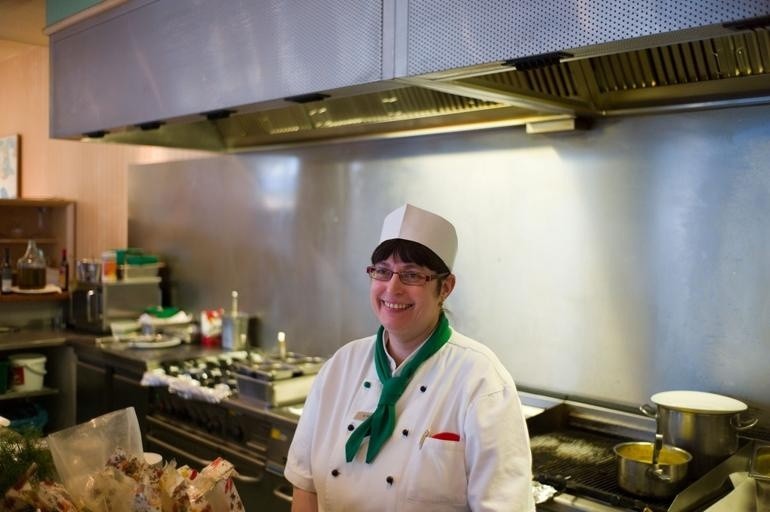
[0,198,78,336]
[1,325,80,438]
[69,361,151,449]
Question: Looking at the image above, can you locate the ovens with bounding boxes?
[144,410,266,512]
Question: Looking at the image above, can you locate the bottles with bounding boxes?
[59,249,71,292]
[2,247,11,293]
[17,240,47,288]
[103,252,117,284]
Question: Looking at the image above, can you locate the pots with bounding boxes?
[638,390,761,478]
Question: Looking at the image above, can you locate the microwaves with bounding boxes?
[71,281,161,333]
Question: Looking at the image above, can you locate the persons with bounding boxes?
[284,203,537,512]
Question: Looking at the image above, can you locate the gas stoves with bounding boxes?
[161,355,255,388]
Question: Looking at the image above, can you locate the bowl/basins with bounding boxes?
[143,450,163,467]
[614,432,693,498]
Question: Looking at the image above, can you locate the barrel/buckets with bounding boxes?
[9,353,47,391]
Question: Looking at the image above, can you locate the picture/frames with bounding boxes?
[0,133,22,200]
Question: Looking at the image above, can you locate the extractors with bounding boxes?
[41,1,769,152]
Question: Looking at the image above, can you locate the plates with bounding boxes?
[12,286,57,293]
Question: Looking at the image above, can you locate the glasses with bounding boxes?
[365,265,451,288]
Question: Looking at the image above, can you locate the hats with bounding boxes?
[376,203,460,274]
